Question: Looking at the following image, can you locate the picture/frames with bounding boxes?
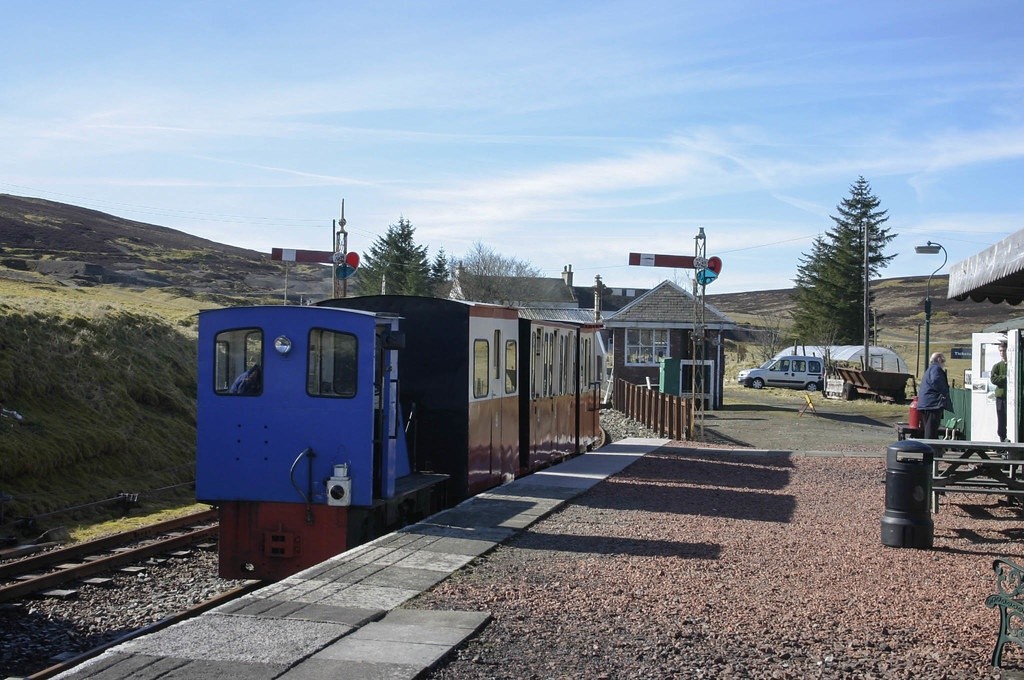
[972,378,988,393]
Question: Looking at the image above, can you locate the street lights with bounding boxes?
[914,241,947,370]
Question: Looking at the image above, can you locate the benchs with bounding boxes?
[955,482,1007,486]
[984,557,1024,667]
[932,486,1024,495]
[937,468,1024,477]
[966,478,998,481]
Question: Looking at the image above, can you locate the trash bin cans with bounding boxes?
[880,440,934,549]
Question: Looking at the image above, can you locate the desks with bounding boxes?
[909,438,1024,513]
[895,422,959,441]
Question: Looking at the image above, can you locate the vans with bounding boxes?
[737,356,825,392]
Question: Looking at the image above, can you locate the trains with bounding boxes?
[194,294,603,580]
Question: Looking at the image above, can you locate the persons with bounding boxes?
[991,341,1006,441]
[918,352,955,439]
[221,364,261,398]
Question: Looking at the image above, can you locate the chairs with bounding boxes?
[943,416,962,440]
